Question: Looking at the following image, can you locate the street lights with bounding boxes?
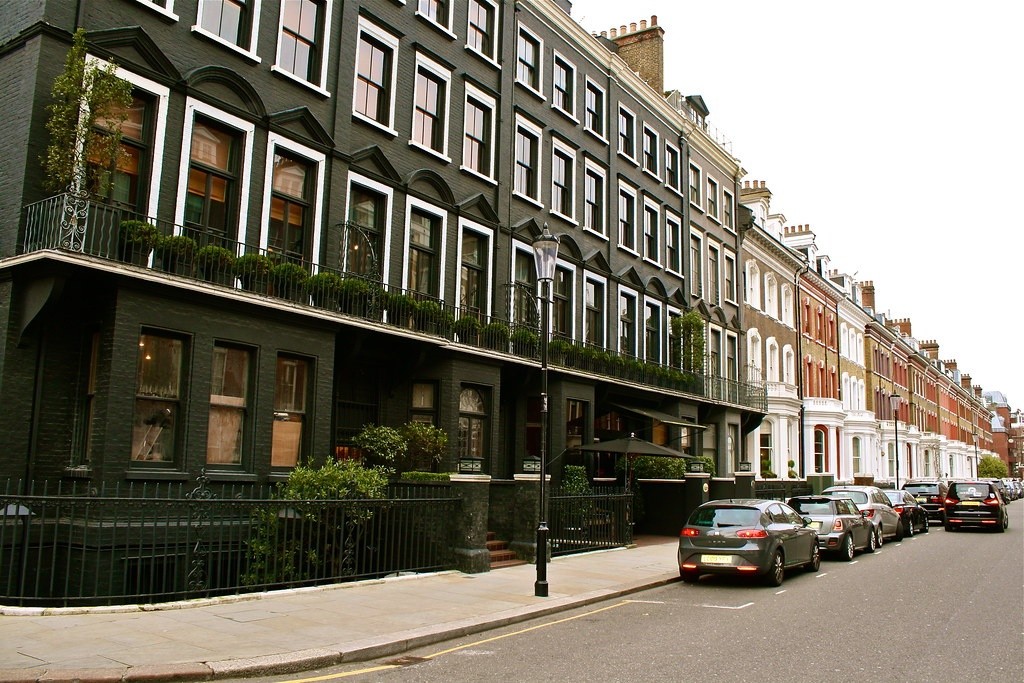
[529,221,561,599]
[971,432,980,479]
[890,391,902,490]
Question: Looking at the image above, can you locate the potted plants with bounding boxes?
[454,316,481,347]
[272,263,308,303]
[371,287,390,321]
[386,294,416,328]
[413,300,440,334]
[117,219,164,266]
[307,271,340,312]
[510,329,538,357]
[239,251,273,295]
[405,421,448,470]
[481,324,511,352]
[352,423,408,476]
[557,463,592,547]
[341,278,371,317]
[548,340,570,366]
[569,345,698,391]
[196,245,235,286]
[438,310,455,341]
[162,235,199,277]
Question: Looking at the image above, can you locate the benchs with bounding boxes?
[756,482,786,503]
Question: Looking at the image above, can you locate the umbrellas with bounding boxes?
[575,432,697,493]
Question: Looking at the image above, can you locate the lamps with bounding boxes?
[522,455,542,473]
[456,451,483,473]
[739,461,752,471]
[688,459,706,472]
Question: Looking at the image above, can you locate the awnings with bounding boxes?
[612,401,707,428]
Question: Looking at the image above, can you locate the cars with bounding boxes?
[880,488,929,537]
[785,494,876,560]
[980,477,1024,504]
[677,498,821,586]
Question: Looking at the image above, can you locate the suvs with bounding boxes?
[820,485,903,549]
[901,479,949,523]
[944,481,1009,533]
